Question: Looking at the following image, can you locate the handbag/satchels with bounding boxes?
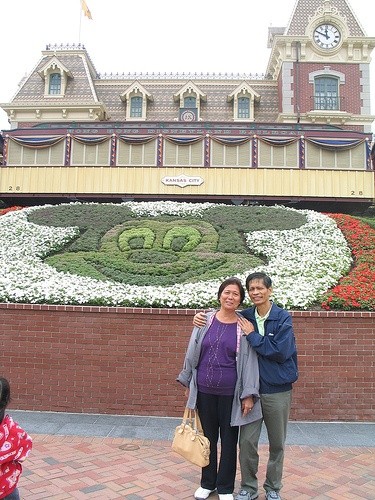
[172,404,210,467]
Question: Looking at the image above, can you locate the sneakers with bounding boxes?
[266,491,281,500]
[234,489,258,500]
[194,486,217,500]
[218,494,233,500]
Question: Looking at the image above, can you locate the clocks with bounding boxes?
[313,23,341,49]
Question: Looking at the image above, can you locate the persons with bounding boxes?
[193,273,298,500]
[176,277,260,500]
[0,377,32,500]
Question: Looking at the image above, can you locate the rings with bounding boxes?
[249,409,252,411]
